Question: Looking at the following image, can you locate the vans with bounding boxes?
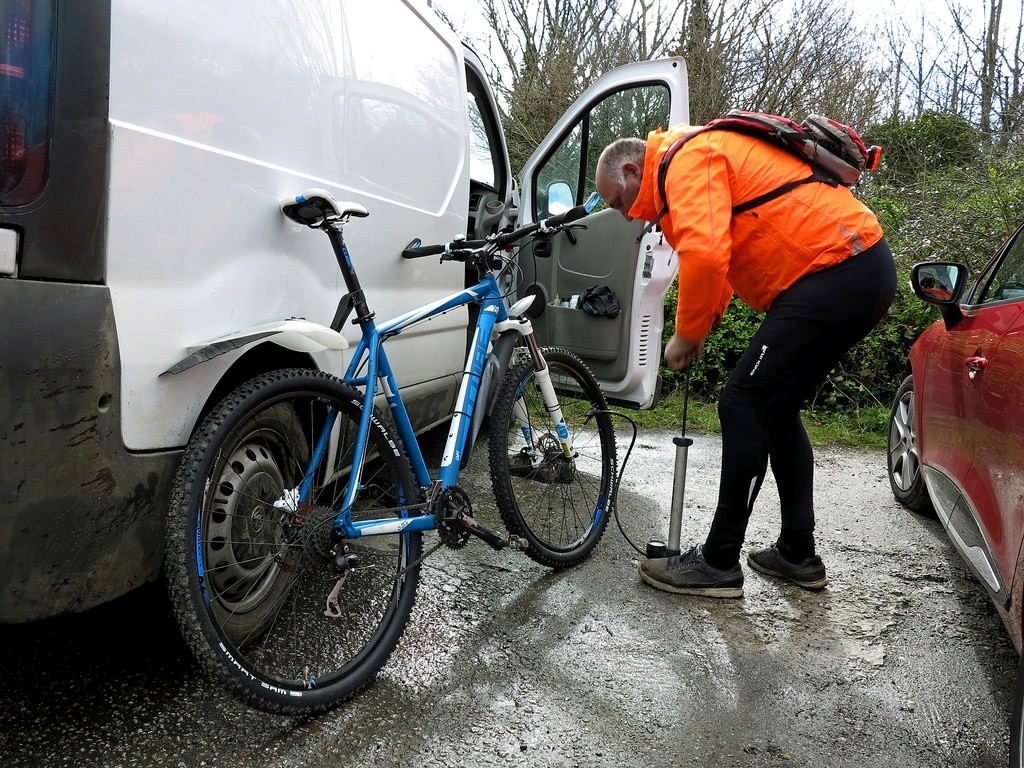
[0,0,691,653]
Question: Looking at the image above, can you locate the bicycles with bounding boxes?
[158,189,620,720]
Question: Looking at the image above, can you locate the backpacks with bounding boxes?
[658,110,882,215]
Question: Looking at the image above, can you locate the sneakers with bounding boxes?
[639,544,744,597]
[748,543,829,589]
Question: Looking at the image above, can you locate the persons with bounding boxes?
[595,124,896,597]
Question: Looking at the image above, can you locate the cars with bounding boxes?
[886,222,1024,664]
